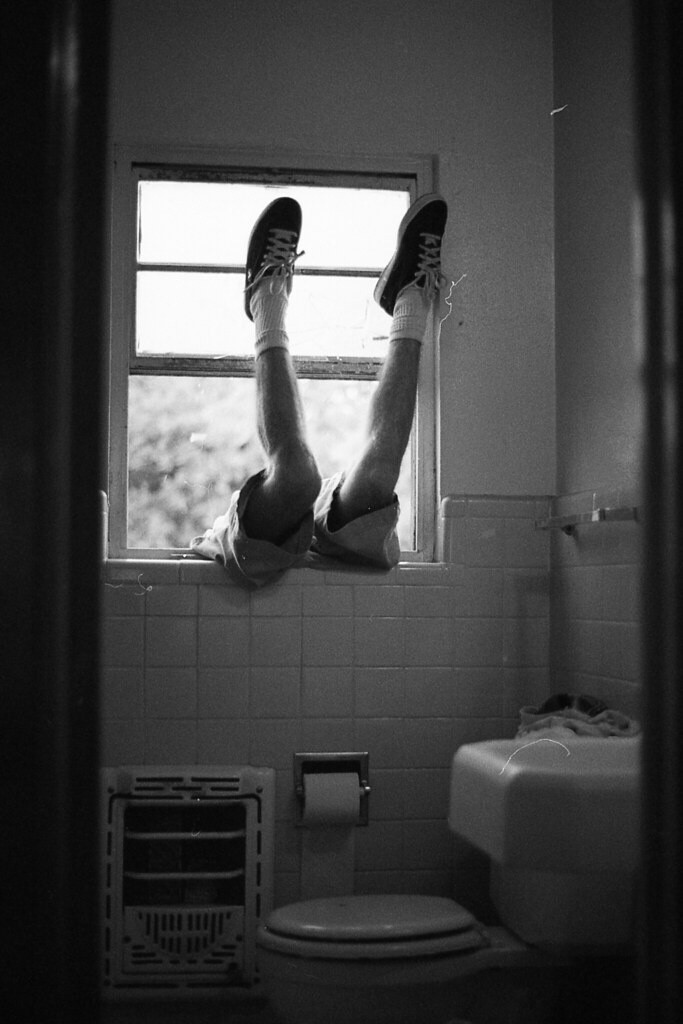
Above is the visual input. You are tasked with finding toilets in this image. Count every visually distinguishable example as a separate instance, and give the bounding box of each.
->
[256,732,646,1021]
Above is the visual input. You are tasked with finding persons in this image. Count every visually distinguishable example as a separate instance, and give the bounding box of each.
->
[189,193,455,590]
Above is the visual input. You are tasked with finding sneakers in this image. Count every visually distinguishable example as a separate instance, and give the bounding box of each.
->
[373,193,448,317]
[243,196,306,322]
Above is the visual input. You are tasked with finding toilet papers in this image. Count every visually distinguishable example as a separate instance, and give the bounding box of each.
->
[302,769,366,829]
